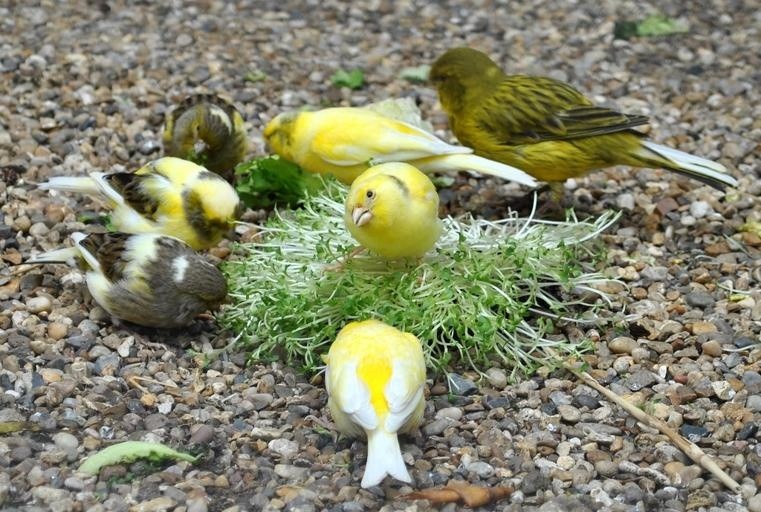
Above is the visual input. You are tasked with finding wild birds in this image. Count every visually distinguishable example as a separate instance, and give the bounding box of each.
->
[341,164,443,265]
[38,151,246,248]
[432,48,741,213]
[161,90,248,183]
[33,233,232,333]
[264,103,542,190]
[327,317,432,489]
